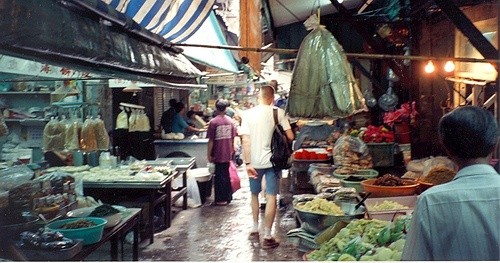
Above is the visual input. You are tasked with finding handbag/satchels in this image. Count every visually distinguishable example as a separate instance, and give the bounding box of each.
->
[229,160,240,193]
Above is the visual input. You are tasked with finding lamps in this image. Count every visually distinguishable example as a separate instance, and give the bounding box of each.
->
[122,80,143,93]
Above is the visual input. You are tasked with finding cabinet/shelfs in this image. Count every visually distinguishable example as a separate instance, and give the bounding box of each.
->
[0,90,81,121]
[154,139,209,168]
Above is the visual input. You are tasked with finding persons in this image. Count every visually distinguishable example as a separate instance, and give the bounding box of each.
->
[399,105,500,262]
[206,99,238,206]
[157,97,253,140]
[267,80,285,111]
[238,85,294,249]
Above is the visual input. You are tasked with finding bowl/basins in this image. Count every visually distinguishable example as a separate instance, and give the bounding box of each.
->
[65,205,127,229]
[294,165,421,235]
[0,215,40,239]
[44,217,107,245]
[191,167,213,182]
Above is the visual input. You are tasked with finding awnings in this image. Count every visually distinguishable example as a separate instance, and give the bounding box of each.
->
[0,0,216,92]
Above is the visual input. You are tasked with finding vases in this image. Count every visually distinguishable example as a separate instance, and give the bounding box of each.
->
[398,144,411,164]
[368,146,394,166]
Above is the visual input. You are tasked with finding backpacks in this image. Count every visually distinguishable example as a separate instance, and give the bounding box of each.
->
[271,109,292,169]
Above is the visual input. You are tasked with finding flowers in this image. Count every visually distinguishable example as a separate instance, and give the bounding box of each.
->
[384,101,416,143]
[361,125,393,143]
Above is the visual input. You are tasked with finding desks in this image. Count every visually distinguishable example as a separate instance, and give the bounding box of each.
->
[3,204,141,262]
[171,171,188,211]
[83,172,176,244]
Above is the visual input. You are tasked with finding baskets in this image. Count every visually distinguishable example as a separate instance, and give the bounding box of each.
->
[416,177,435,195]
[332,169,378,179]
[361,177,420,196]
[341,177,370,193]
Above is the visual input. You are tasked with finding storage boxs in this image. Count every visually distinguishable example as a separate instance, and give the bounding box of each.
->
[292,147,432,221]
[15,239,82,261]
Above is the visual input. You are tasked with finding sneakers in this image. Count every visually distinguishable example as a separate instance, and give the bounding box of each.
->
[263,237,279,248]
[248,232,260,241]
